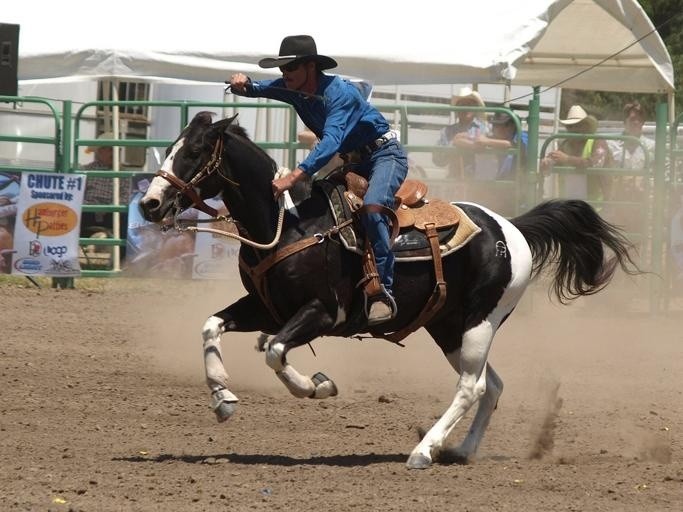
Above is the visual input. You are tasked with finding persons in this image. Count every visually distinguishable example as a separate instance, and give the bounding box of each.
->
[71,132,135,268]
[603,103,657,198]
[539,103,610,198]
[431,87,493,180]
[472,106,530,182]
[228,34,410,328]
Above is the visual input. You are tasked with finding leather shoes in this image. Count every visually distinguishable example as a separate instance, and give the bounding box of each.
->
[368,292,393,324]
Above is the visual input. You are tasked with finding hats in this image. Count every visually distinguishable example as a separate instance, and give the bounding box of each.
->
[451,87,486,118]
[488,112,513,124]
[258,35,338,71]
[556,105,598,134]
[85,132,114,153]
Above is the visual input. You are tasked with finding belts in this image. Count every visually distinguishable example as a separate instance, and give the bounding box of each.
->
[339,130,397,163]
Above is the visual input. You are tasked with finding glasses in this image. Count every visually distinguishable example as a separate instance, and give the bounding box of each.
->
[278,60,305,72]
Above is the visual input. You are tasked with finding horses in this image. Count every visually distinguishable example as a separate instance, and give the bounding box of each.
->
[137,111,667,469]
